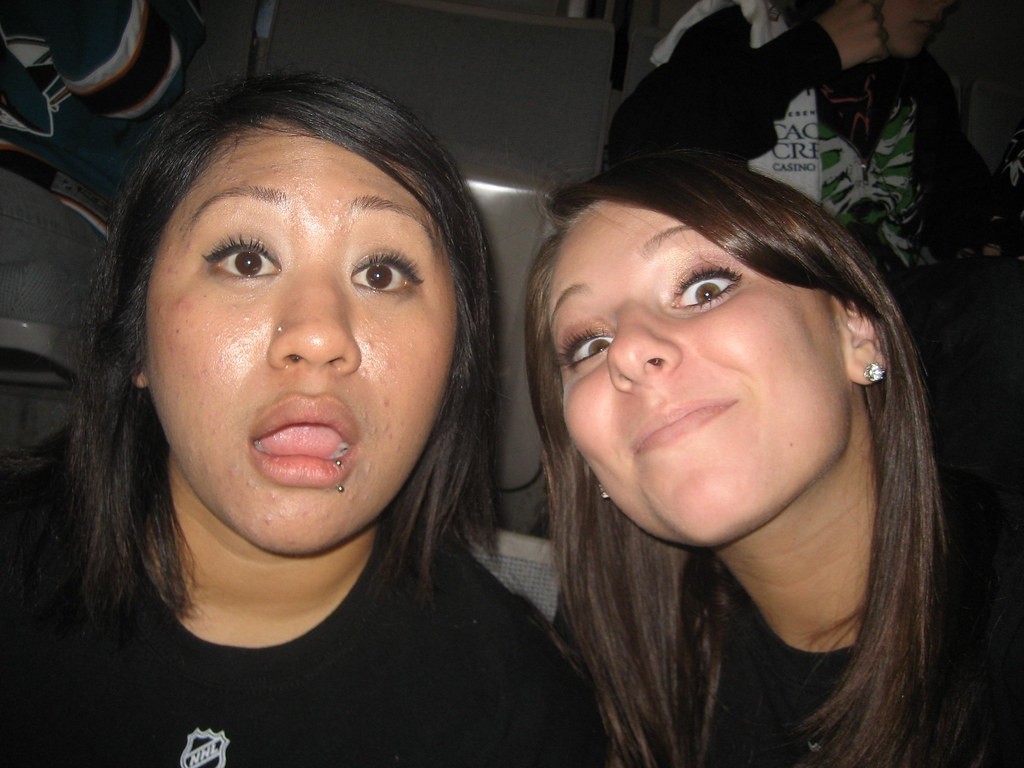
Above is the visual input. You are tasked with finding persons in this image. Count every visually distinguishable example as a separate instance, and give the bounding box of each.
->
[610,0,1023,487]
[1,69,609,768]
[526,157,1023,768]
